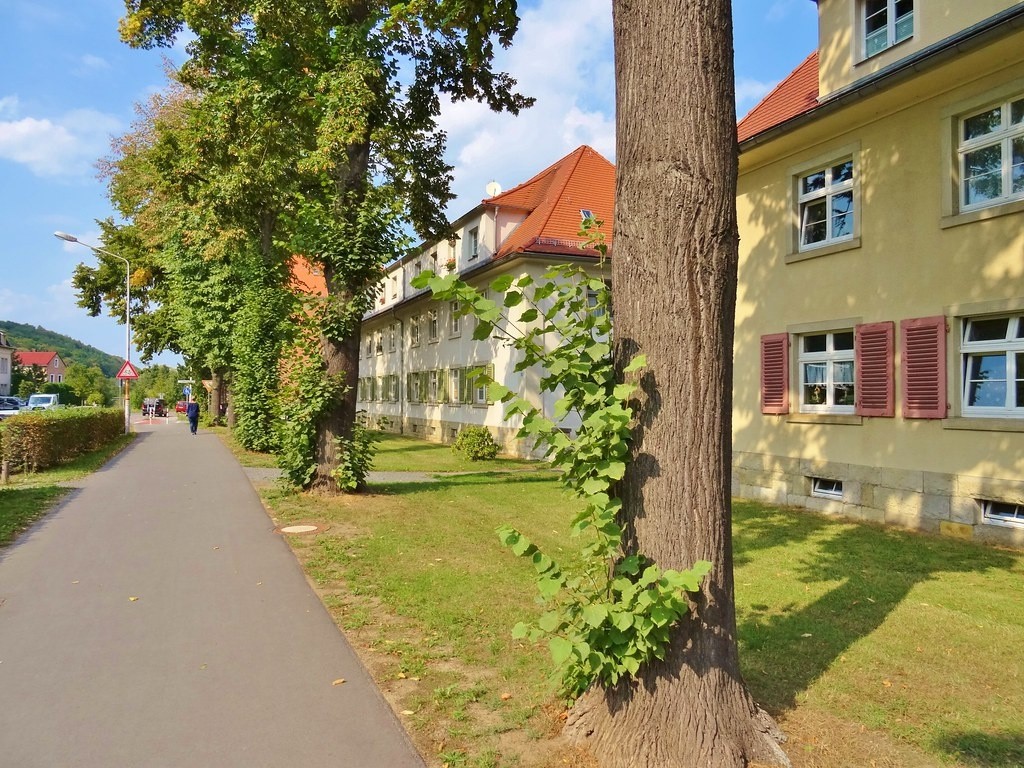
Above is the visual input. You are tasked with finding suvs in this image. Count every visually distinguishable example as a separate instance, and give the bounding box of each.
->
[176,401,186,412]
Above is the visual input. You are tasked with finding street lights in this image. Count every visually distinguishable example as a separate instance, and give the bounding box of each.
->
[53,231,130,435]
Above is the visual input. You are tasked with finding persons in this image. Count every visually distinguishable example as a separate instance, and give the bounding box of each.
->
[185,395,200,435]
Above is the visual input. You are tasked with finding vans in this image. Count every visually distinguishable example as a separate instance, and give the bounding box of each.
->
[27,393,58,412]
[143,398,167,417]
[0,397,26,410]
